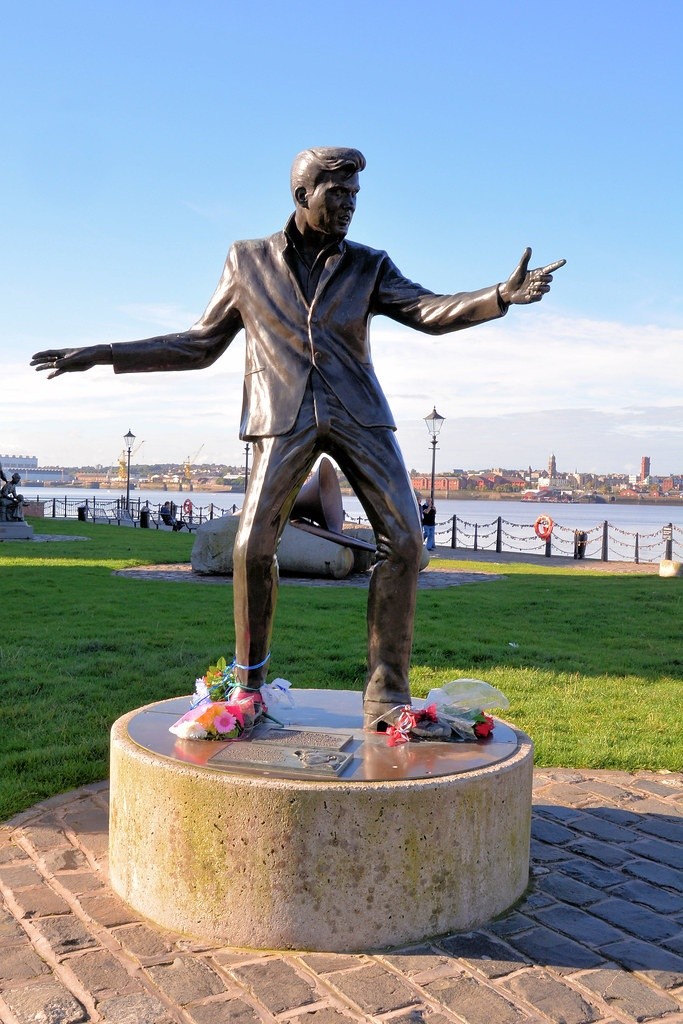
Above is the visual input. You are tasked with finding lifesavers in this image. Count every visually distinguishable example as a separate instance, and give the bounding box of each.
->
[183,498,193,513]
[533,516,554,539]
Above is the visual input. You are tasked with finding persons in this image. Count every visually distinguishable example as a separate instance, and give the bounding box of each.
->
[0,466,25,523]
[417,496,437,551]
[28,148,566,737]
[160,502,185,533]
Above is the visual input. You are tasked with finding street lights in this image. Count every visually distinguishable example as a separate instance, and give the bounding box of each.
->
[423,406,448,507]
[123,429,137,510]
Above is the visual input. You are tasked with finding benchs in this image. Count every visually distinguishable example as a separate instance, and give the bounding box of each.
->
[87,507,117,525]
[182,515,208,533]
[111,508,140,528]
[150,512,185,533]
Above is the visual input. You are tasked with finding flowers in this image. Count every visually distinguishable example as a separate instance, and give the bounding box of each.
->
[421,703,494,741]
[190,656,235,709]
[169,694,284,742]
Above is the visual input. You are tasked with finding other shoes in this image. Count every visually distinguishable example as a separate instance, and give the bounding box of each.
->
[427,549,434,551]
